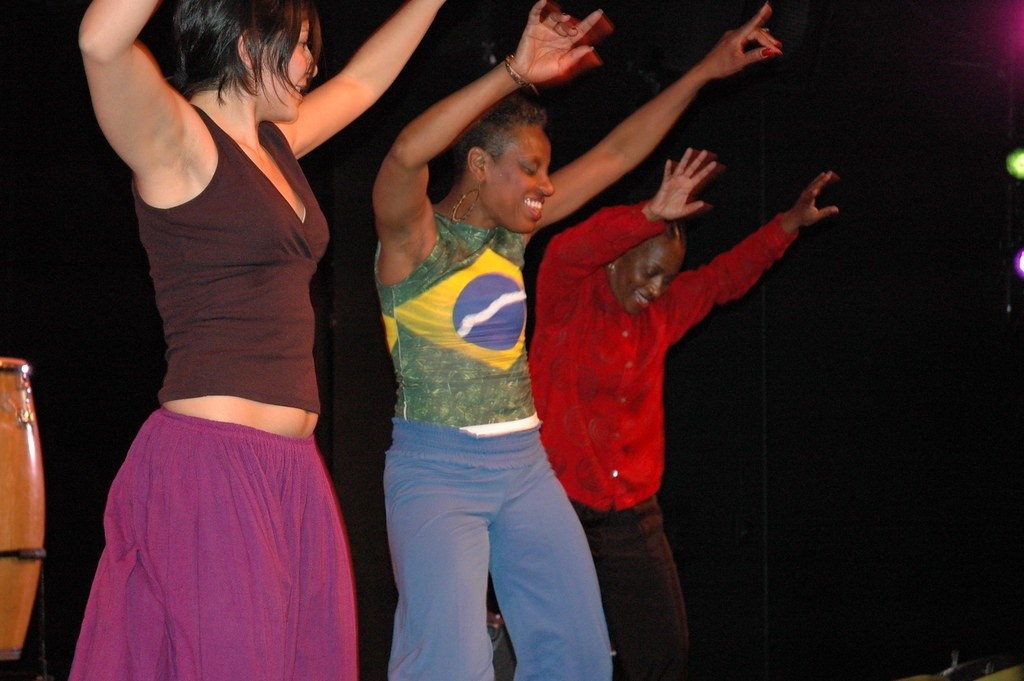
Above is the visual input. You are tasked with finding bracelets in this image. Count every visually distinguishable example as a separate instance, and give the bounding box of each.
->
[504,54,541,97]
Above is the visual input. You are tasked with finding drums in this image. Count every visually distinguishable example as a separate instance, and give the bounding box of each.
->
[1,356,50,663]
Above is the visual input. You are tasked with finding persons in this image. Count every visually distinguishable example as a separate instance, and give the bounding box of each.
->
[68,0,448,681]
[528,145,843,681]
[370,0,787,681]
